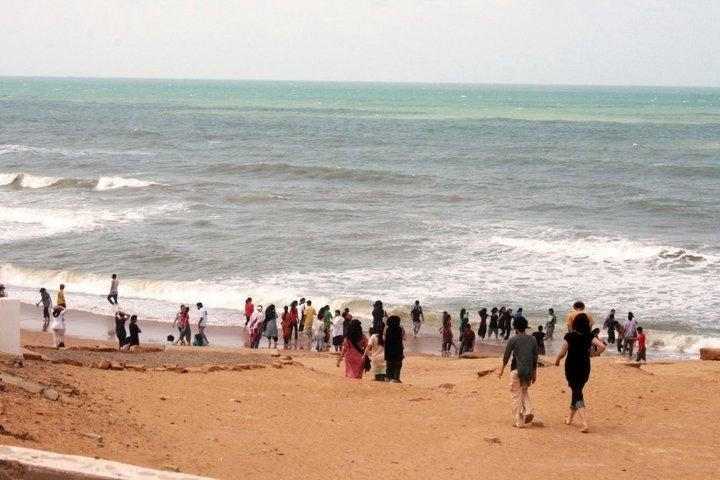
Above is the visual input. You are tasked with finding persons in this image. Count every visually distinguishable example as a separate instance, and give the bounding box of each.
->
[383,315,406,382]
[591,309,645,362]
[128,315,142,346]
[57,284,67,309]
[115,311,130,349]
[51,306,67,348]
[336,319,368,379]
[458,308,475,355]
[361,321,387,381]
[478,306,522,341]
[534,325,549,355]
[410,299,424,338]
[107,276,119,305]
[556,313,606,433]
[544,308,557,339]
[372,300,388,333]
[172,302,209,344]
[313,305,352,353]
[498,316,539,429]
[440,312,459,356]
[38,288,53,325]
[244,297,317,349]
[567,302,594,332]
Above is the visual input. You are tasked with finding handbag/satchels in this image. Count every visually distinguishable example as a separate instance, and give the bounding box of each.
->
[365,356,372,373]
